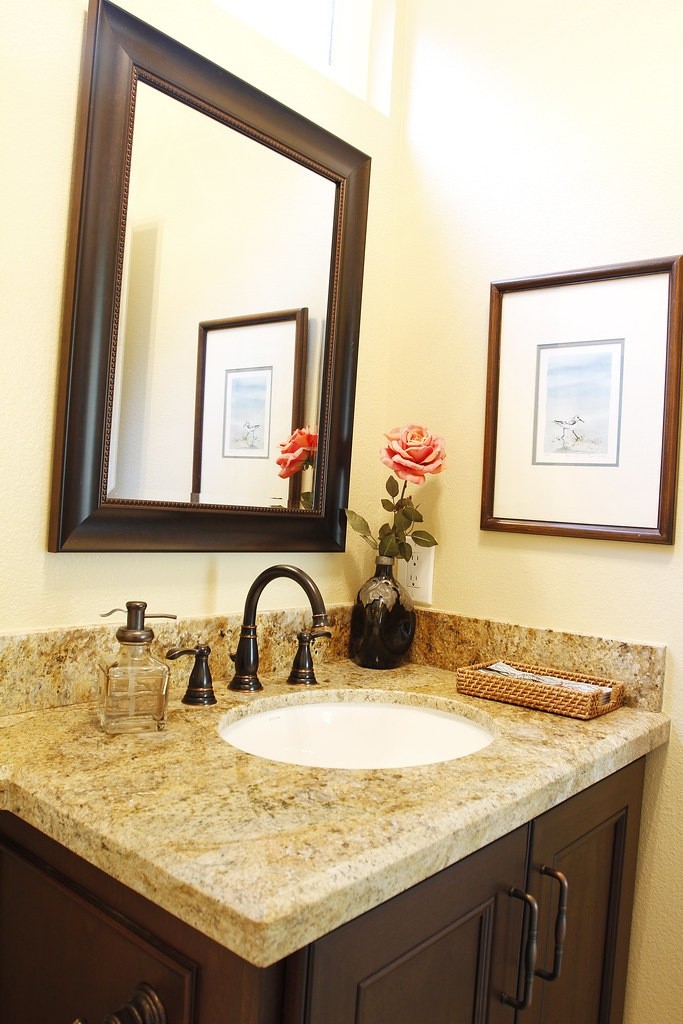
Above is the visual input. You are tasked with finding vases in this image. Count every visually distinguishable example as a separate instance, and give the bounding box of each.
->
[349,556,417,669]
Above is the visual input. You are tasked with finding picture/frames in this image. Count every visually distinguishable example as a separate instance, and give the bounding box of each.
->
[480,255,683,546]
[191,307,308,509]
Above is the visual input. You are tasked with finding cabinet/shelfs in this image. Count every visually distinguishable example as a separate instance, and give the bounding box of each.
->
[283,754,648,1024]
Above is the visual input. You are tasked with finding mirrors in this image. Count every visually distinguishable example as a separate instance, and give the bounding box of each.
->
[48,1,372,552]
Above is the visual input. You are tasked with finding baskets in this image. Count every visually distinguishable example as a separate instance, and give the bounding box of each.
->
[456,660,625,719]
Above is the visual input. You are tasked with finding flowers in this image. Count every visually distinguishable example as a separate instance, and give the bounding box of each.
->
[344,425,446,563]
[271,425,319,510]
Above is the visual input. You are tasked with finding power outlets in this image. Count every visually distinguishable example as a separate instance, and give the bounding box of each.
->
[397,535,436,604]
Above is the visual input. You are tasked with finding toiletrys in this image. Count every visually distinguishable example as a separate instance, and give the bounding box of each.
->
[96,601,177,734]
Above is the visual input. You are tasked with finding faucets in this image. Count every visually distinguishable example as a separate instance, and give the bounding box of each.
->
[226,564,330,692]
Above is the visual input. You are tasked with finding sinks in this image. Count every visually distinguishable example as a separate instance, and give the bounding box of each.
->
[209,688,503,769]
